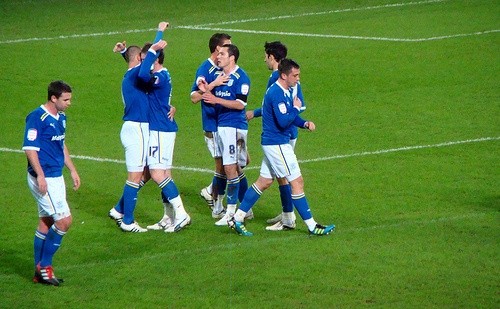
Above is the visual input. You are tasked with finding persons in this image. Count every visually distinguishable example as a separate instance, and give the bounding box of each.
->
[22,80,82,285]
[106,21,177,233]
[112,39,192,233]
[189,33,250,214]
[196,44,254,225]
[226,59,336,236]
[246,40,306,231]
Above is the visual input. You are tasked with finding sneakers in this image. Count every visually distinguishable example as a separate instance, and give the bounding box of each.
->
[308,224,335,236]
[120,219,148,232]
[147,215,174,230]
[211,207,227,219]
[227,217,254,236]
[200,188,215,211]
[33,276,63,287]
[164,213,191,234]
[265,220,296,231]
[266,214,283,223]
[108,208,125,221]
[214,213,234,225]
[244,211,254,220]
[36,260,60,286]
